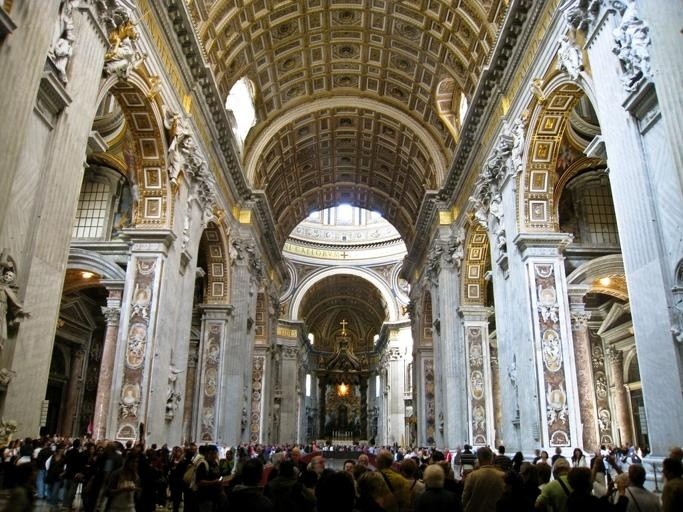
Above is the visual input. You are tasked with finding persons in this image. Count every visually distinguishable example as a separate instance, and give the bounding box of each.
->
[1,438,683,512]
